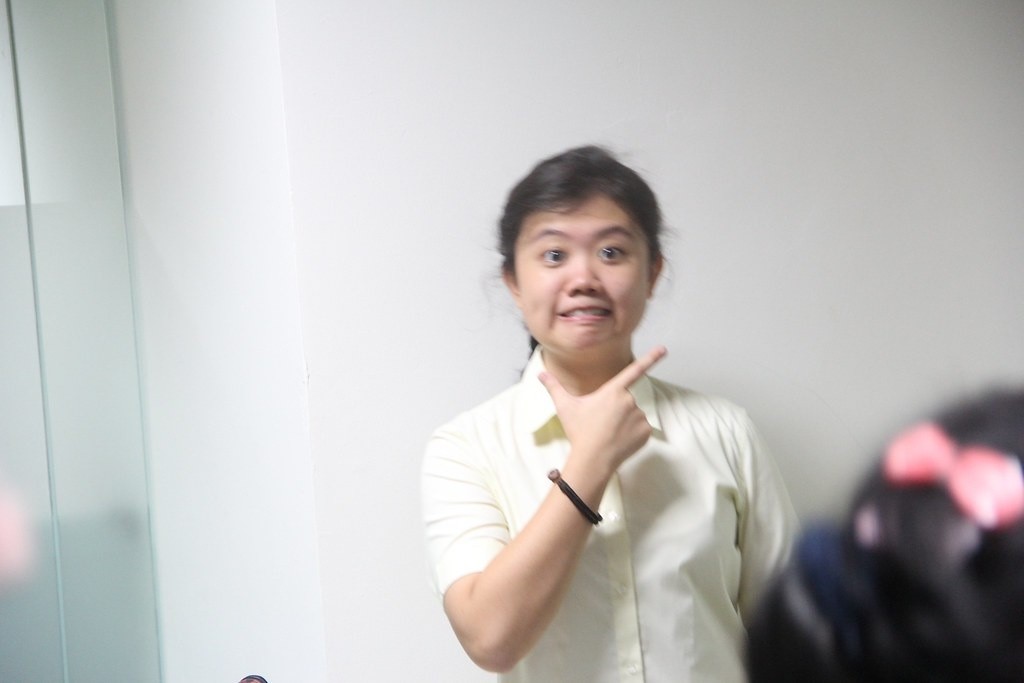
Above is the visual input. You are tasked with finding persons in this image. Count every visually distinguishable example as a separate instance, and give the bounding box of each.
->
[422,145,803,682]
[744,389,1024,680]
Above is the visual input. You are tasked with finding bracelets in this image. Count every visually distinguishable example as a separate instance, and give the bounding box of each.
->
[548,471,603,526]
